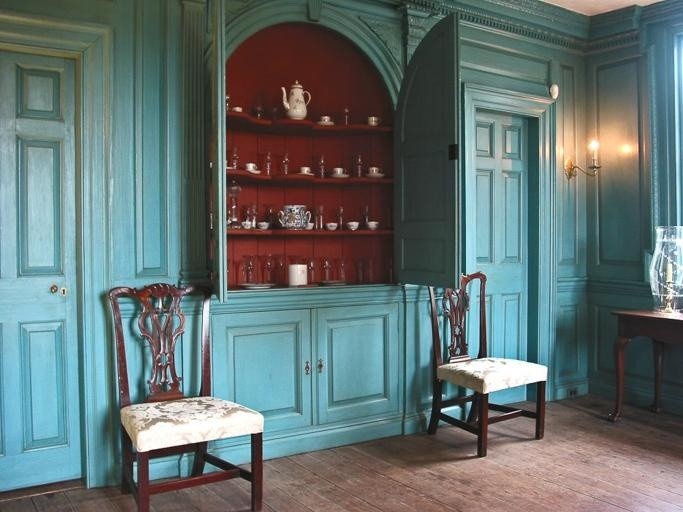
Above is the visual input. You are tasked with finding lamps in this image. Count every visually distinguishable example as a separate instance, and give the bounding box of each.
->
[561,133,606,185]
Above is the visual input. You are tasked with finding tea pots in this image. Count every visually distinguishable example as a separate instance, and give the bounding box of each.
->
[280,79,312,120]
[278,204,311,229]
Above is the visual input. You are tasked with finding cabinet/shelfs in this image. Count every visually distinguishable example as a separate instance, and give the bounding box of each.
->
[404,298,470,437]
[213,297,407,473]
[223,96,401,294]
[111,304,213,483]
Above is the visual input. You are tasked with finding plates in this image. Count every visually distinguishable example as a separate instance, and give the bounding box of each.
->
[366,173,384,178]
[317,121,336,126]
[239,283,276,290]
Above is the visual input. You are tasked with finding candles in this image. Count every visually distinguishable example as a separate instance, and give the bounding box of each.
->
[664,256,675,283]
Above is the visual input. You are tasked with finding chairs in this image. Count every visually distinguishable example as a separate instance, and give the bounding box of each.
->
[426,267,549,461]
[104,281,266,511]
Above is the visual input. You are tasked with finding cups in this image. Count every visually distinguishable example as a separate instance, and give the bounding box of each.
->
[232,107,242,113]
[368,167,381,174]
[241,222,378,230]
[367,116,381,127]
[241,254,349,285]
[320,116,331,123]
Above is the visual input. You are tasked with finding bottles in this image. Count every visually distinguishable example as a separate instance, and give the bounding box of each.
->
[243,206,257,229]
[335,205,345,230]
[360,205,369,230]
[229,146,366,178]
[225,180,241,231]
[344,105,352,125]
[316,205,325,228]
[253,96,265,119]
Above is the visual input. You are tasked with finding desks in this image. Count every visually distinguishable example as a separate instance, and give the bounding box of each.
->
[603,304,683,421]
